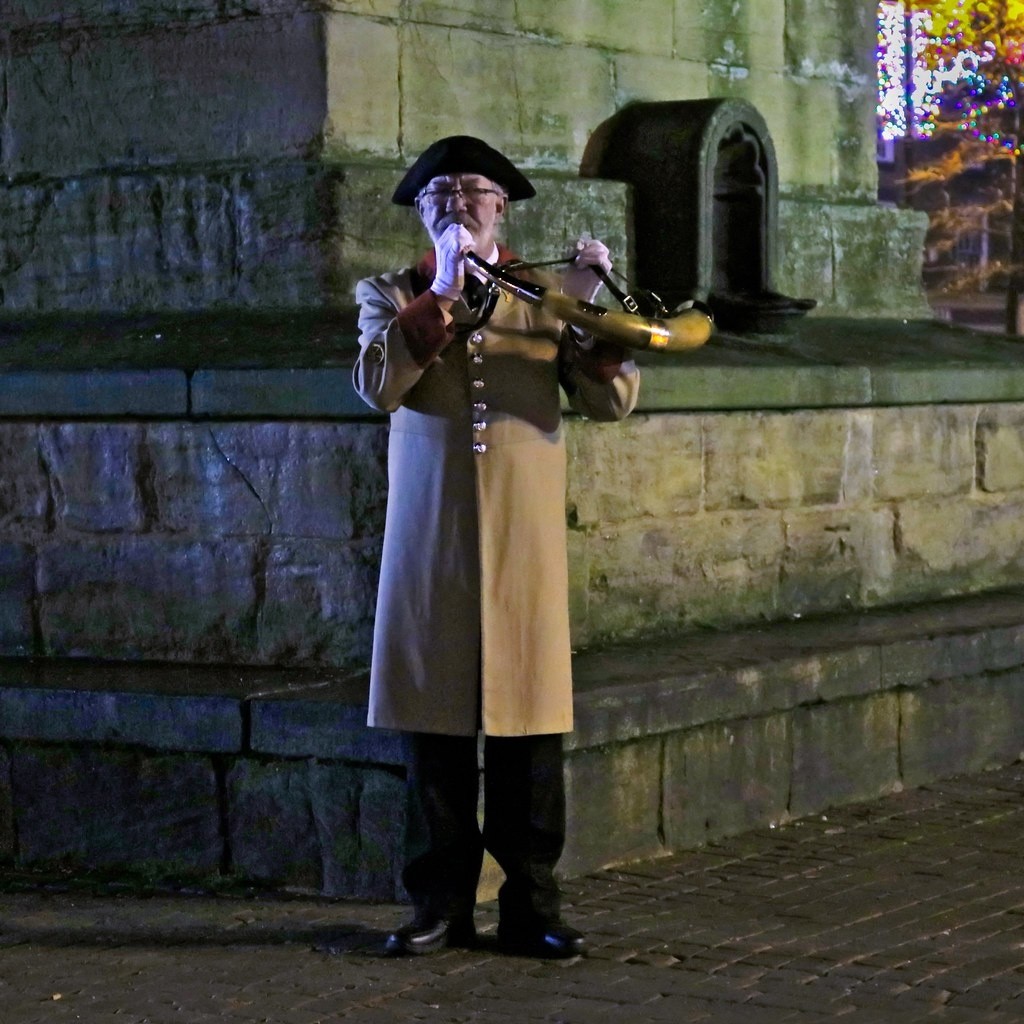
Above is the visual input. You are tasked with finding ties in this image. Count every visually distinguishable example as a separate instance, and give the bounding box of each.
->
[464,272,482,308]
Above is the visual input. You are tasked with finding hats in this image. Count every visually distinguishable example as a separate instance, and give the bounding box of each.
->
[391,136,536,207]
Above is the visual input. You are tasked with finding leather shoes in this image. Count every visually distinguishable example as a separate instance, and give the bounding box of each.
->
[386,911,477,955]
[496,923,586,957]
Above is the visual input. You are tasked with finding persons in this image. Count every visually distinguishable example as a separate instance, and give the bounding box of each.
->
[352,134,641,958]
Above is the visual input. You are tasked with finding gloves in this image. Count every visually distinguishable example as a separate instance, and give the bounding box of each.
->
[430,223,478,302]
[562,239,614,305]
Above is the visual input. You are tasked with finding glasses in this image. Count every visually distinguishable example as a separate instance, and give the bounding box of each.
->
[421,186,502,205]
